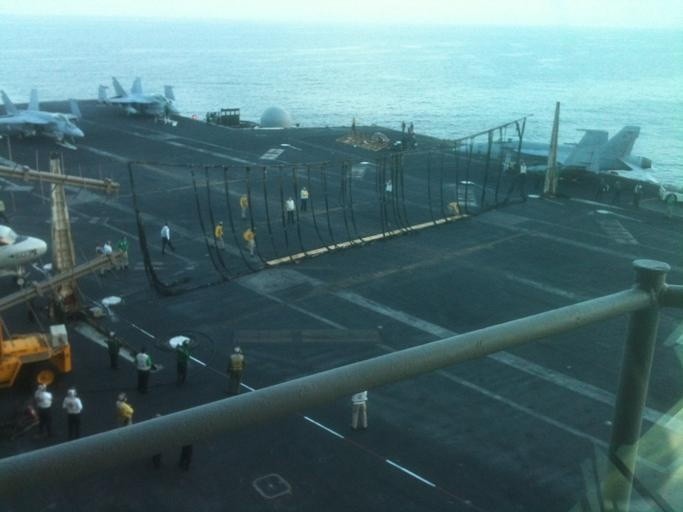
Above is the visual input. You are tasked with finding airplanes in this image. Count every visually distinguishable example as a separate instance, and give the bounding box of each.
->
[450,123,660,190]
[99,75,178,122]
[0,88,85,154]
[0,201,48,290]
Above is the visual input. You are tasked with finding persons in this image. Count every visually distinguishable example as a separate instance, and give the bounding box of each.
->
[34,381,53,433]
[178,446,193,470]
[612,179,624,204]
[148,411,165,466]
[631,182,645,209]
[118,234,129,270]
[103,240,112,267]
[59,390,84,439]
[133,344,150,393]
[160,220,175,254]
[597,174,607,196]
[115,389,133,426]
[285,196,295,223]
[214,220,224,251]
[243,225,258,256]
[347,389,370,430]
[238,190,248,220]
[298,185,310,210]
[105,331,120,365]
[93,242,105,278]
[175,342,186,386]
[226,345,244,396]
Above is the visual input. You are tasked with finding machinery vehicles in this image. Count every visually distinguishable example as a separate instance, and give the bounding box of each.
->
[0,243,129,388]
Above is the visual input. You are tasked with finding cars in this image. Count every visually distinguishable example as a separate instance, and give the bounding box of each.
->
[659,181,683,207]
